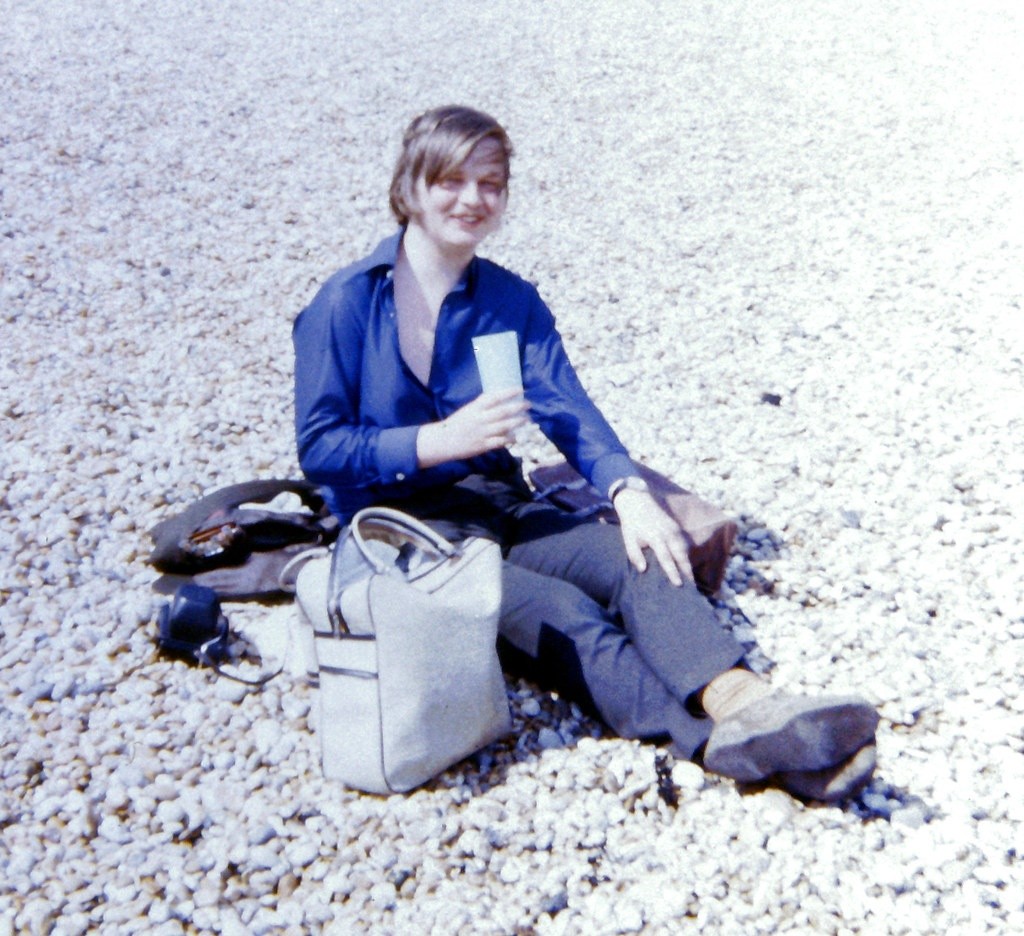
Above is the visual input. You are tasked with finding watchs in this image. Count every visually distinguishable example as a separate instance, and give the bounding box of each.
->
[611,476,649,504]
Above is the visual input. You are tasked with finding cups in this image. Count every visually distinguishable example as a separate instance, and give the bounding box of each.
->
[473,330,530,446]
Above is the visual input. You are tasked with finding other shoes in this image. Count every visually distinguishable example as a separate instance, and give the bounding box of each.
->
[701,688,877,804]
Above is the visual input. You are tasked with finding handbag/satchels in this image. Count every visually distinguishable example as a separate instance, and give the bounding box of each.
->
[278,506,515,796]
[149,481,330,571]
[529,453,736,600]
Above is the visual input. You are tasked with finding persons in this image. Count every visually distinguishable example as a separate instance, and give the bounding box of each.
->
[291,104,882,803]
